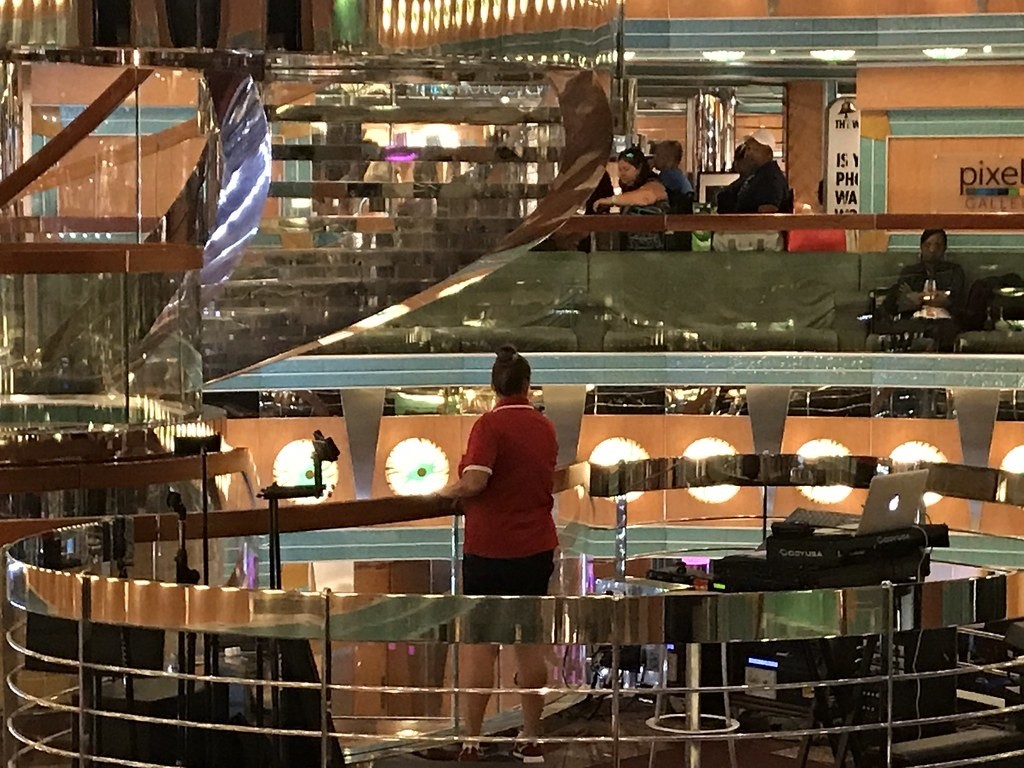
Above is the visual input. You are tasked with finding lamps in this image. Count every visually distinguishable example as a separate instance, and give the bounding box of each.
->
[998,443,1024,510]
[793,437,854,505]
[588,436,652,504]
[683,437,743,504]
[273,438,339,506]
[385,436,448,498]
[888,440,951,510]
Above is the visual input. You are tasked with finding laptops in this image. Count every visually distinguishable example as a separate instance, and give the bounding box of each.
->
[813,469,930,539]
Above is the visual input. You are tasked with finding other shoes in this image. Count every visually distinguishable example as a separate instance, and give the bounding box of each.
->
[513,737,545,763]
[458,742,480,763]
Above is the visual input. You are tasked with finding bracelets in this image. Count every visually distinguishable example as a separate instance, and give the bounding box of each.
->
[611,195,617,204]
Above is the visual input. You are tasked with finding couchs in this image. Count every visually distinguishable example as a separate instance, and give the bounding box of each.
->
[587,251,860,354]
[859,250,1024,356]
[318,250,588,355]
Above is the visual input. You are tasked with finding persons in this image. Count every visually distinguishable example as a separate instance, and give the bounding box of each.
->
[884,227,968,354]
[736,129,788,215]
[715,143,753,216]
[429,345,559,764]
[649,141,694,207]
[590,145,672,251]
[316,131,523,282]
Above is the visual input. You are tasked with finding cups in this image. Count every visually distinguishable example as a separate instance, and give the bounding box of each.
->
[923,279,936,300]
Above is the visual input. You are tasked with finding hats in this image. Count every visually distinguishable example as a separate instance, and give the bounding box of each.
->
[744,129,776,153]
